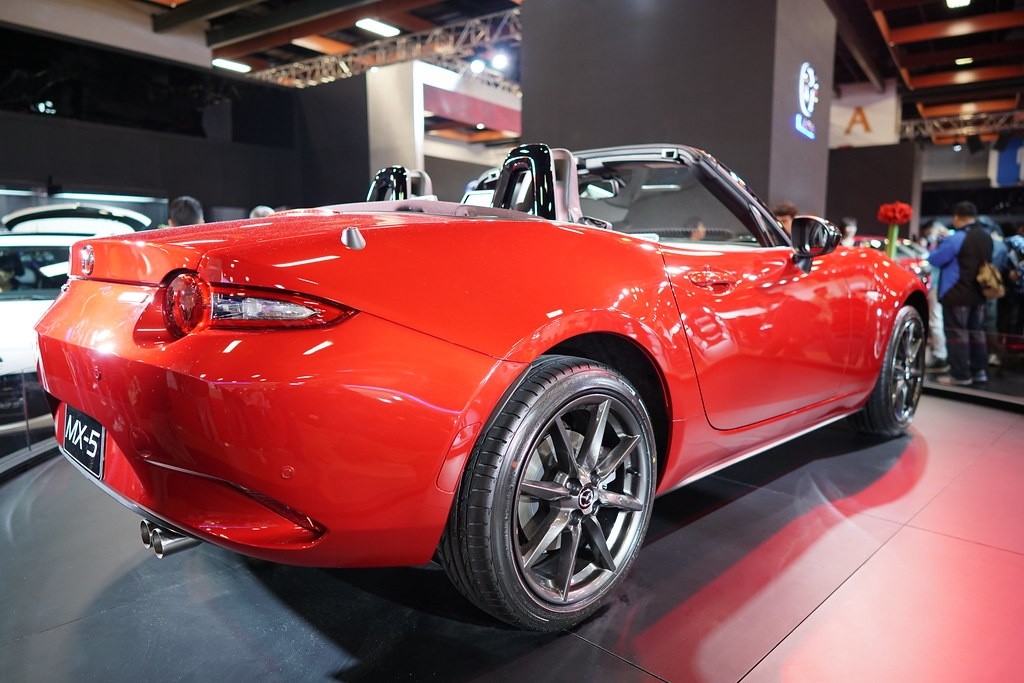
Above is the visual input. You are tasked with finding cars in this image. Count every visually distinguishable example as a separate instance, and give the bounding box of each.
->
[0,204,153,446]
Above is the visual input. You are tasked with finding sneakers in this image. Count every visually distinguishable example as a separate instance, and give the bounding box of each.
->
[936,373,972,385]
[972,369,988,381]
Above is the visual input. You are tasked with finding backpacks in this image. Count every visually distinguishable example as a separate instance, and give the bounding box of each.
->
[975,260,1004,298]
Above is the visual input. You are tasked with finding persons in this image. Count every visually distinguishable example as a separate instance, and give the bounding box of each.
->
[0,257,34,293]
[926,202,995,385]
[913,215,1024,348]
[681,217,707,242]
[773,201,797,237]
[975,215,1009,366]
[836,217,860,247]
[169,195,205,227]
[922,219,957,375]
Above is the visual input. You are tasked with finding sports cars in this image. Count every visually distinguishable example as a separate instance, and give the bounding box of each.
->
[36,141,931,636]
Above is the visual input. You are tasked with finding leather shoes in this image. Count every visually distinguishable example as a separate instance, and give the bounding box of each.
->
[924,358,951,373]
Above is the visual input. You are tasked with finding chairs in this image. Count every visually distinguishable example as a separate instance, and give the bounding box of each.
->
[365,164,433,204]
[488,140,583,224]
